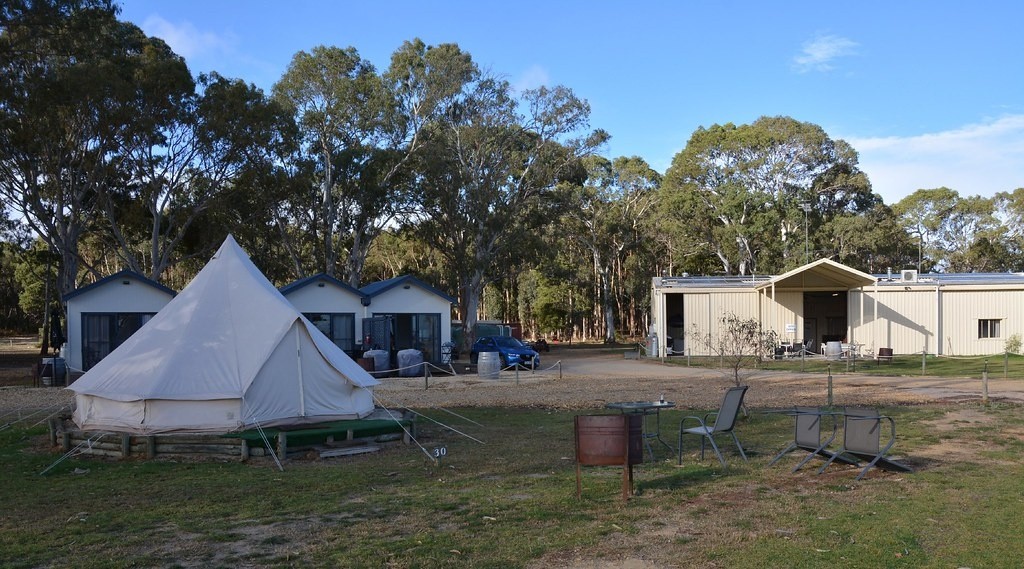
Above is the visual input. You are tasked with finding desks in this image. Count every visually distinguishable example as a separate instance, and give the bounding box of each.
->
[781,344,791,358]
[605,399,676,459]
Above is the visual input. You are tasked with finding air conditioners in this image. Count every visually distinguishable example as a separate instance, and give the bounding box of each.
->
[902,269,918,283]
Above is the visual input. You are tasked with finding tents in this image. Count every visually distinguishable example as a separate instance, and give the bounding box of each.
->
[63,232,383,434]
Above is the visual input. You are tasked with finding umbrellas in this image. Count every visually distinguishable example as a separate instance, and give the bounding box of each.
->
[50,308,66,353]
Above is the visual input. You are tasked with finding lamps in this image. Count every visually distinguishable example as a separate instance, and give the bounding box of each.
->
[319,282,324,287]
[122,279,130,285]
[404,285,411,289]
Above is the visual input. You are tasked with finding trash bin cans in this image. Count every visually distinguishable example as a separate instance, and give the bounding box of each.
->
[775,348,784,359]
[646,337,659,357]
[478,351,501,380]
[42,358,65,386]
[826,341,842,361]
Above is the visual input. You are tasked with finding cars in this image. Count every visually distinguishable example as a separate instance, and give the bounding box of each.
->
[470,335,540,371]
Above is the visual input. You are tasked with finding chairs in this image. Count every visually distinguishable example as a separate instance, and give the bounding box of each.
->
[793,340,803,357]
[780,338,792,357]
[758,404,864,473]
[816,408,914,485]
[798,339,814,357]
[674,386,754,478]
